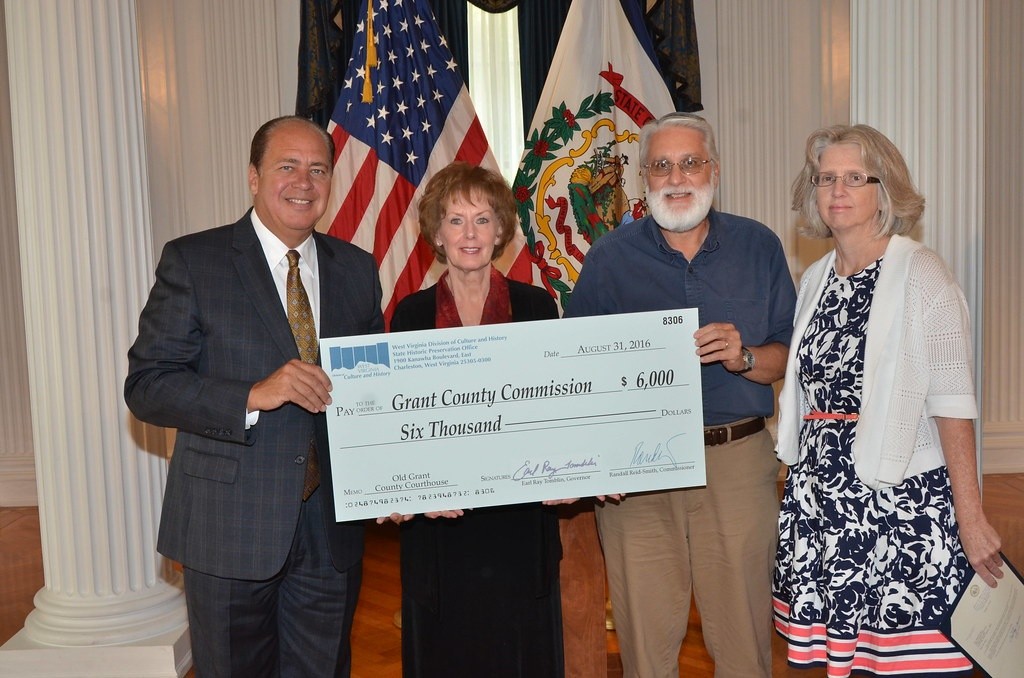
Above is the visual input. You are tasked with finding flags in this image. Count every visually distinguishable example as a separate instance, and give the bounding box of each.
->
[511,0,677,318]
[313,0,534,333]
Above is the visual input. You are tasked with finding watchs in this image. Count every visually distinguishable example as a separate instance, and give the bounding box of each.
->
[727,344,757,375]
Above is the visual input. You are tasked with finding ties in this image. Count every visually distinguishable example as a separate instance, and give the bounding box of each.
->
[287,248,322,500]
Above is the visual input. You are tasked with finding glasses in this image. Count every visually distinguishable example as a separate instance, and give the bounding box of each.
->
[644,158,713,177]
[811,172,881,187]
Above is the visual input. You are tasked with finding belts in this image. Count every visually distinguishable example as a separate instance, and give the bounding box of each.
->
[704,414,768,447]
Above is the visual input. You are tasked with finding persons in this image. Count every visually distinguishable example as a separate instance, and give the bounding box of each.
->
[769,121,1004,677]
[387,160,569,678]
[562,114,796,676]
[121,117,380,678]
